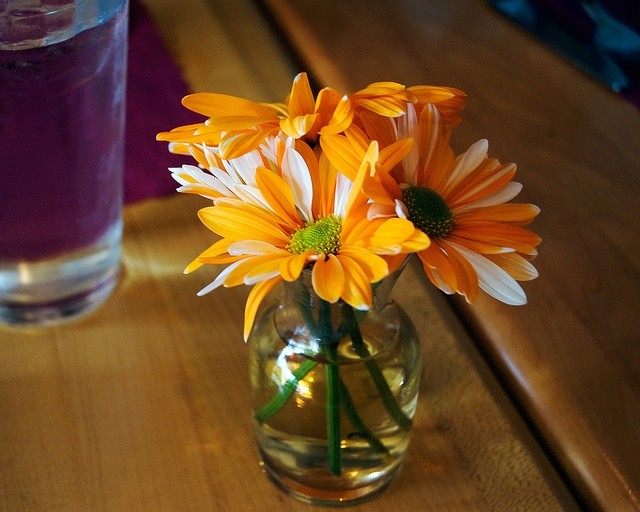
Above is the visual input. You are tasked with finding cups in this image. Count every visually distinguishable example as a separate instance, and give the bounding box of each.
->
[0,0,124,331]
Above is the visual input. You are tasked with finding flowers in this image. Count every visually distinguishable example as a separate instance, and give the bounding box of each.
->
[157,71,553,475]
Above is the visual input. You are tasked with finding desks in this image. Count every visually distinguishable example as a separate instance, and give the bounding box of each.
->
[0,0,640,512]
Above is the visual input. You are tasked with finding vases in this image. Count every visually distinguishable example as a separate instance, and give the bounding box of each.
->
[248,262,421,508]
